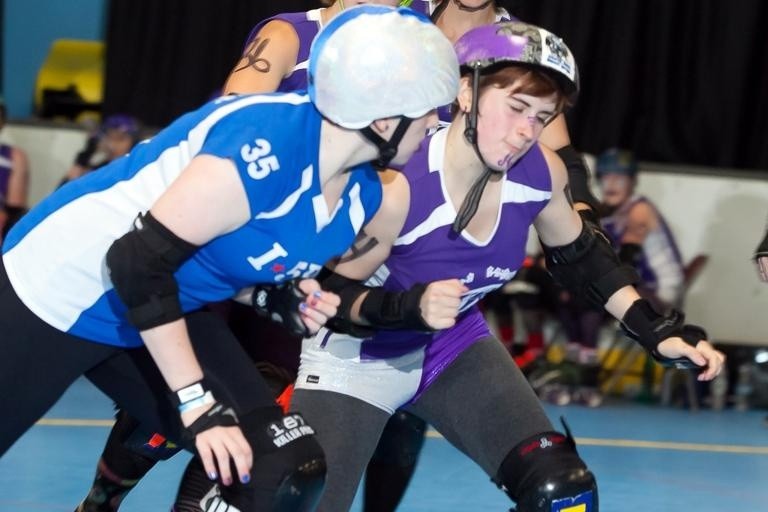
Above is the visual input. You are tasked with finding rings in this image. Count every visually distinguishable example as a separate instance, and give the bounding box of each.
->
[759,270,765,273]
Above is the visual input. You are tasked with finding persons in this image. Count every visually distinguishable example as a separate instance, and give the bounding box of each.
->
[286,21,724,512]
[77,0,410,510]
[537,146,684,399]
[487,270,550,380]
[57,116,142,193]
[361,0,599,512]
[2,141,28,233]
[753,227,768,283]
[1,0,457,512]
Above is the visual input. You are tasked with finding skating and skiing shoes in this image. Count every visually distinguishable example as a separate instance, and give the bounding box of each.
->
[533,359,603,408]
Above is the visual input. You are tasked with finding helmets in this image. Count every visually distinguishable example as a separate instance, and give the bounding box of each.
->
[596,149,636,178]
[99,116,139,139]
[308,6,458,130]
[454,20,579,100]
